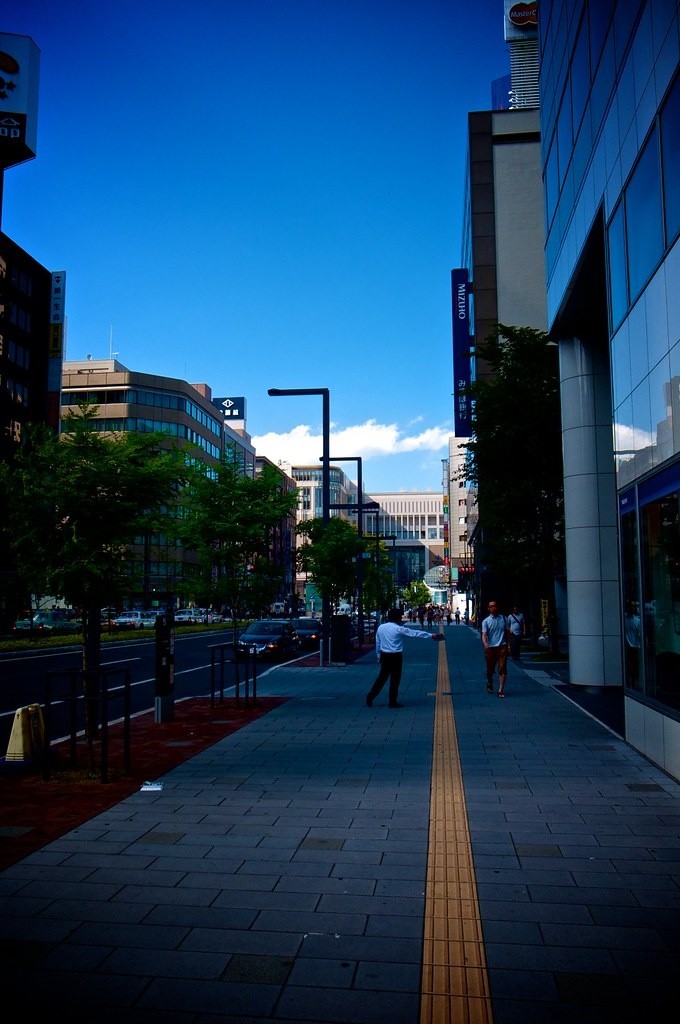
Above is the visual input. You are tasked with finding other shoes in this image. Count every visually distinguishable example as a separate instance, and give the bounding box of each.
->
[389,704,404,708]
[366,696,372,707]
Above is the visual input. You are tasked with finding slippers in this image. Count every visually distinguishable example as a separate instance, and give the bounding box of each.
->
[487,681,493,693]
[498,692,505,698]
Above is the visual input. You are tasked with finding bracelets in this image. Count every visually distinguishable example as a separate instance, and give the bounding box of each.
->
[508,646,510,648]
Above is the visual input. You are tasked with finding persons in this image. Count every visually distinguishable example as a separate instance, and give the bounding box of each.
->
[625,598,680,658]
[366,608,447,709]
[311,607,319,618]
[482,599,511,698]
[506,606,525,661]
[408,602,469,629]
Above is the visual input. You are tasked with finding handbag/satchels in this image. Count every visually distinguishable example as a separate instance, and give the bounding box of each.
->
[521,624,524,632]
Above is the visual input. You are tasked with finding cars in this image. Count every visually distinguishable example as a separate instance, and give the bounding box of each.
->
[112,608,237,628]
[298,607,306,615]
[291,618,323,651]
[336,607,350,615]
[12,611,80,630]
[238,620,302,662]
